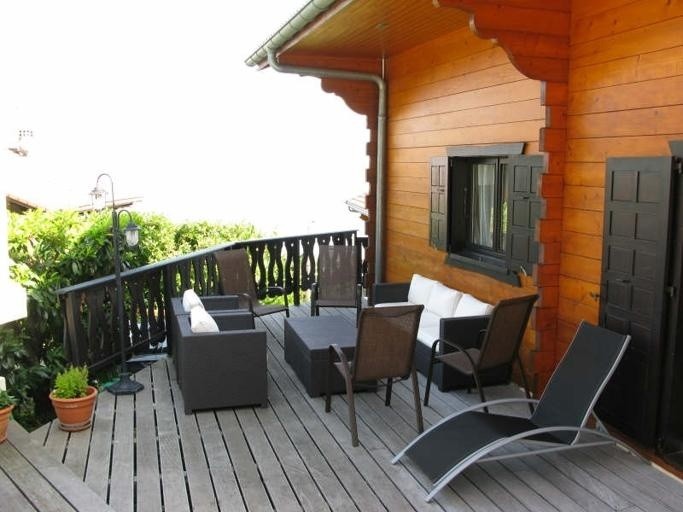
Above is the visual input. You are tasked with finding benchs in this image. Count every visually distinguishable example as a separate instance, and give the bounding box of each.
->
[367,273,514,392]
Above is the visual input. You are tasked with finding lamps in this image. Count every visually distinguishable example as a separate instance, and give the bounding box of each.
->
[117,207,138,247]
[89,174,117,211]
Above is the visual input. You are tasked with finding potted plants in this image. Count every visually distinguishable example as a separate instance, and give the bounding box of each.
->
[0,389,16,443]
[50,361,98,431]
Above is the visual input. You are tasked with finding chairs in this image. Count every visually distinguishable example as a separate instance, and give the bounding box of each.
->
[424,293,539,414]
[392,320,657,503]
[310,244,362,327]
[169,294,250,383]
[213,249,289,330]
[325,305,424,447]
[170,312,266,415]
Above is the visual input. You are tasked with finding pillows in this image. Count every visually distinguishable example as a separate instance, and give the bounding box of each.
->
[406,274,440,302]
[426,284,460,319]
[190,306,219,333]
[182,289,204,312]
[454,294,494,318]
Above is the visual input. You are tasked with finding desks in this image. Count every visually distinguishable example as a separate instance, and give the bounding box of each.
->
[284,315,378,397]
